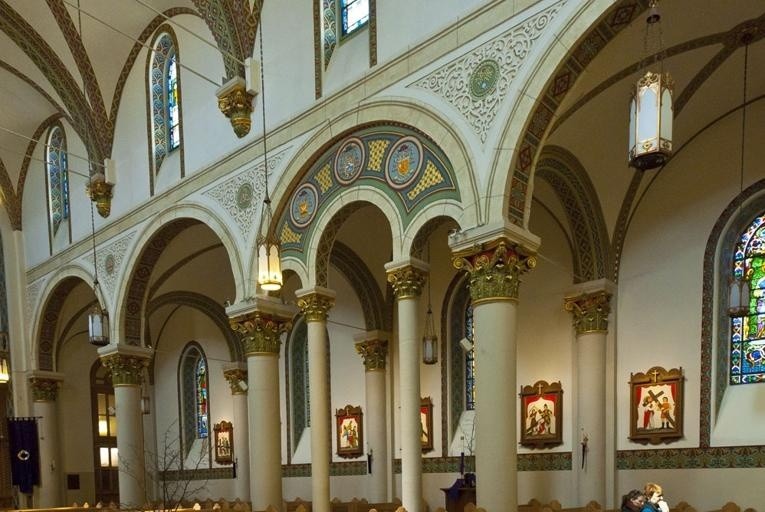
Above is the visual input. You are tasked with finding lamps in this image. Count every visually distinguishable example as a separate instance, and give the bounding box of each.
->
[256,0,283,292]
[421,239,439,366]
[88,1,108,345]
[628,1,675,170]
[726,30,752,318]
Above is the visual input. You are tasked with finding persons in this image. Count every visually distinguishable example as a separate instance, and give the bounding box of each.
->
[659,396,673,428]
[217,437,229,456]
[540,402,553,433]
[341,420,358,448]
[644,397,659,428]
[641,483,670,512]
[528,407,541,435]
[621,488,644,512]
